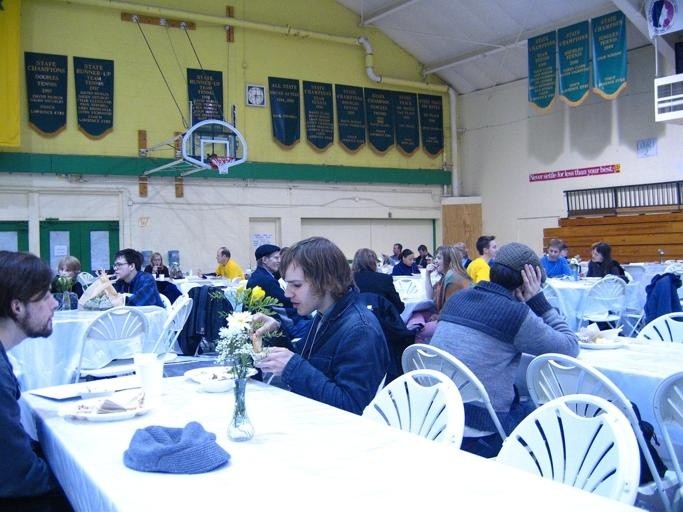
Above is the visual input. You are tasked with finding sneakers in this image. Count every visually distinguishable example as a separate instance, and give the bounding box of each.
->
[408,324,423,336]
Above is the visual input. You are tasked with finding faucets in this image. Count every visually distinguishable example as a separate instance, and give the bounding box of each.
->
[657,248,664,265]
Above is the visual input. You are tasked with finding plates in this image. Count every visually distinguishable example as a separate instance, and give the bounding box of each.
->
[179,276,223,284]
[182,365,257,393]
[578,334,626,349]
[546,277,601,284]
[72,399,149,422]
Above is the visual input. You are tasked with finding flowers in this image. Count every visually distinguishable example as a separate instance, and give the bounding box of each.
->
[58,270,73,291]
[657,247,665,255]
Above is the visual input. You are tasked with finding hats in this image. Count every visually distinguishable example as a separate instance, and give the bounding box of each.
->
[122,420,231,474]
[254,244,281,261]
[494,241,547,284]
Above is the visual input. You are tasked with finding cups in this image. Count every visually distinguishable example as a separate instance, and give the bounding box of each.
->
[151,272,165,279]
[133,352,170,398]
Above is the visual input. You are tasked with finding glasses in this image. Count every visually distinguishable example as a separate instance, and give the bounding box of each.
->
[112,262,128,270]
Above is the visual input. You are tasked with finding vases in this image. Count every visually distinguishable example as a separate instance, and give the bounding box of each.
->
[659,255,664,264]
[62,290,71,311]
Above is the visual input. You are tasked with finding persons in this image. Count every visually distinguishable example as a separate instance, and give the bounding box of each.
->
[205,247,245,280]
[243,236,391,416]
[381,237,579,344]
[104,248,165,307]
[587,241,629,336]
[0,250,60,512]
[144,252,170,278]
[351,248,406,314]
[429,242,578,459]
[51,255,84,310]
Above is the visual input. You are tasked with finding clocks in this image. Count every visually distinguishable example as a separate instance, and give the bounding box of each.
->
[246,84,266,107]
